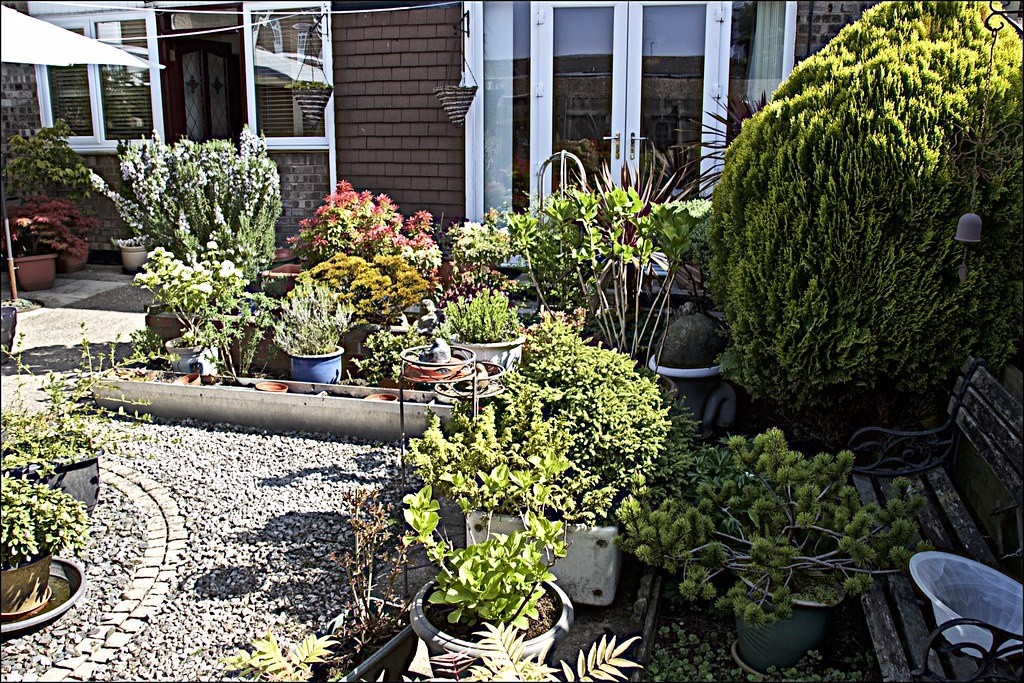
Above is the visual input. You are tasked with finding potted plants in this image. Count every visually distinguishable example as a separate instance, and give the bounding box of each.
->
[0,116,179,635]
[433,40,478,129]
[284,79,335,131]
[324,185,938,683]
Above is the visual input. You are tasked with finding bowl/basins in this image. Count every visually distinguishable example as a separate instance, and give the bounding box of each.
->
[173,373,200,385]
[909,551,1023,659]
[365,393,398,402]
[255,382,289,393]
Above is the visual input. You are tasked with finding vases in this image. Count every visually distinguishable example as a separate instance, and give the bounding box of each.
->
[261,263,302,298]
[272,249,300,265]
[165,335,218,375]
[289,344,345,383]
[107,366,414,404]
[119,240,147,273]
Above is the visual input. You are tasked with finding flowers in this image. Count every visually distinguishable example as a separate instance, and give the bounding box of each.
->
[87,120,608,355]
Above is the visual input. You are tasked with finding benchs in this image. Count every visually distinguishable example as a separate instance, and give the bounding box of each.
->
[833,352,1024,683]
